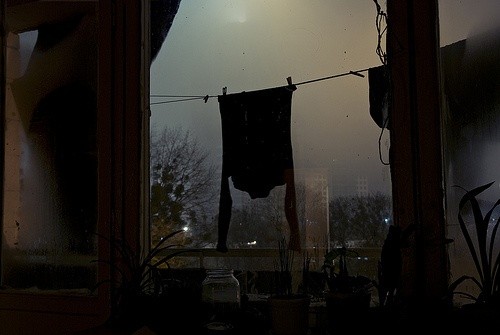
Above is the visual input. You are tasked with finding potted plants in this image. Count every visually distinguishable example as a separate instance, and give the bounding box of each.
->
[86,230,185,335]
[266,237,310,335]
[328,246,371,312]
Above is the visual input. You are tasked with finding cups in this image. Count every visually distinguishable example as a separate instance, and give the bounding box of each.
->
[202,269,240,330]
